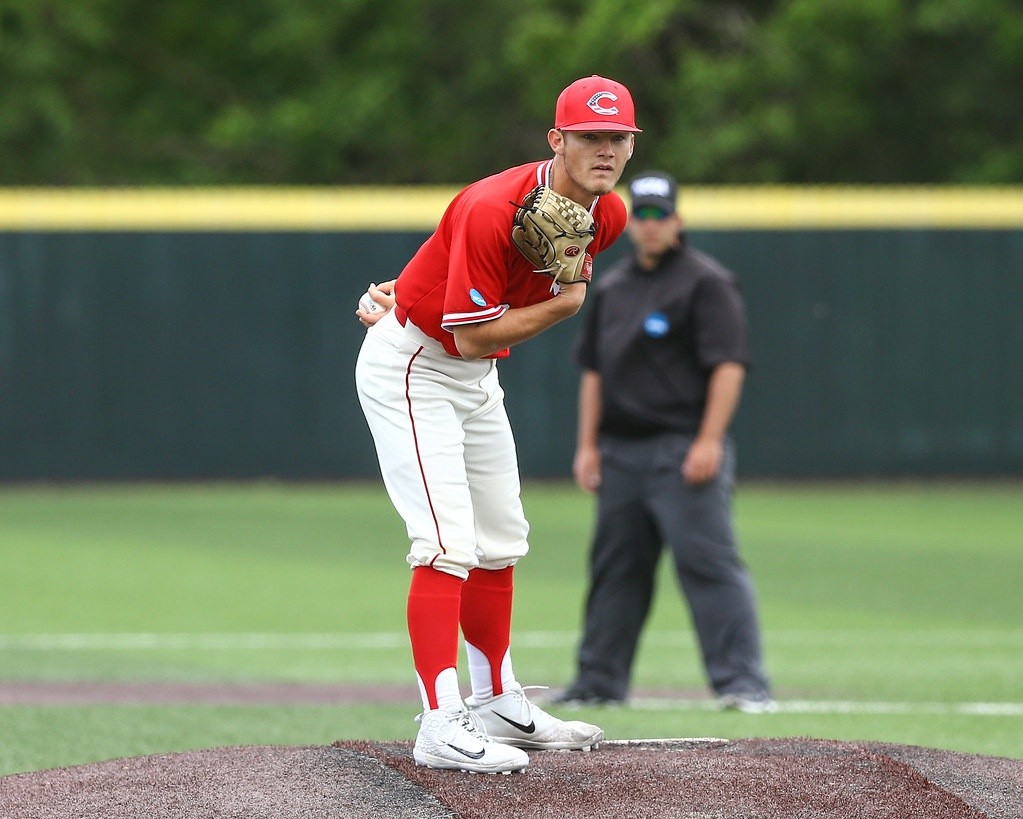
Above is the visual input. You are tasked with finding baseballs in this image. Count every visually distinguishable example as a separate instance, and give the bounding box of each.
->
[359,290,387,315]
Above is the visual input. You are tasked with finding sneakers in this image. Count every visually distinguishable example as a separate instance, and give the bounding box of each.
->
[412,705,529,776]
[464,683,604,752]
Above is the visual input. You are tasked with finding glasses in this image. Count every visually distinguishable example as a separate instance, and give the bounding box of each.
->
[632,206,668,220]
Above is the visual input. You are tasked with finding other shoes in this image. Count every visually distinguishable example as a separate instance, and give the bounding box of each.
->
[533,684,621,708]
[718,684,778,713]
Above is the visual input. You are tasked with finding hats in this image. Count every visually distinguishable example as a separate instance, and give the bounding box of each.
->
[554,75,643,133]
[628,169,677,215]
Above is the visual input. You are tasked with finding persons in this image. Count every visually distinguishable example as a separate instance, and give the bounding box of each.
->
[355,72,637,773]
[531,173,779,716]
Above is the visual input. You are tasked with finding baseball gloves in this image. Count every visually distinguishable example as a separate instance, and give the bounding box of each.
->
[506,183,600,295]
